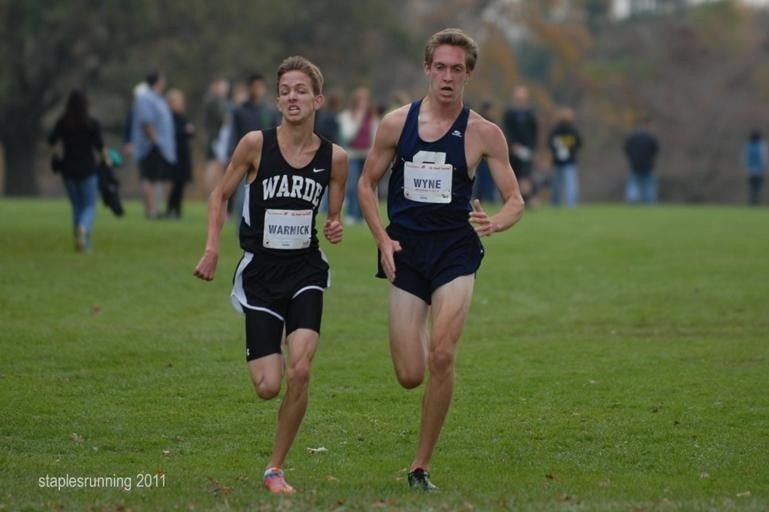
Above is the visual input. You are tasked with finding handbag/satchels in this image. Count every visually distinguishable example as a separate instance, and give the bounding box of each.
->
[96,165,125,217]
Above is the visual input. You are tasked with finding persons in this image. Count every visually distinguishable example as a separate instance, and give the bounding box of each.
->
[194,57,348,492]
[624,112,658,203]
[124,75,581,221]
[51,87,113,252]
[744,127,768,205]
[356,31,526,493]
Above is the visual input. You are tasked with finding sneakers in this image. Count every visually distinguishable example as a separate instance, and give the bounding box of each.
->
[261,467,298,495]
[143,208,181,222]
[406,467,439,491]
[69,226,88,252]
